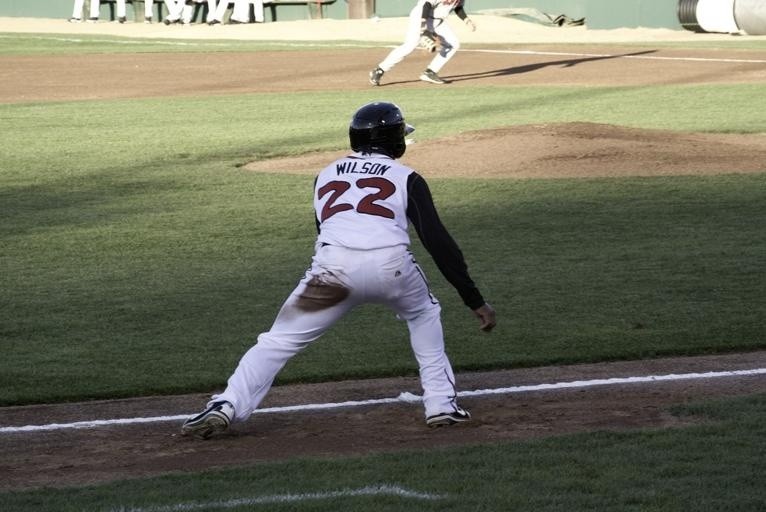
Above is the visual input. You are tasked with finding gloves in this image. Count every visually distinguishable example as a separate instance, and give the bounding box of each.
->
[467,21,477,32]
[476,303,496,329]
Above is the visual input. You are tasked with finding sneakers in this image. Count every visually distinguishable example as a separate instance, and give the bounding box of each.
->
[419,69,445,84]
[368,68,383,85]
[181,401,235,441]
[427,406,471,429]
[69,17,240,25]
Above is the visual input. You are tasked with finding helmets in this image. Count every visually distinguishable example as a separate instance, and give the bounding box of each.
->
[349,101,416,152]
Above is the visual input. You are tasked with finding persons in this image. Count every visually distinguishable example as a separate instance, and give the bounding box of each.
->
[66,1,264,27]
[368,1,478,87]
[181,102,500,437]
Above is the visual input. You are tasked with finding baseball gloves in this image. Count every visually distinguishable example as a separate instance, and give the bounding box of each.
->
[419,31,441,54]
